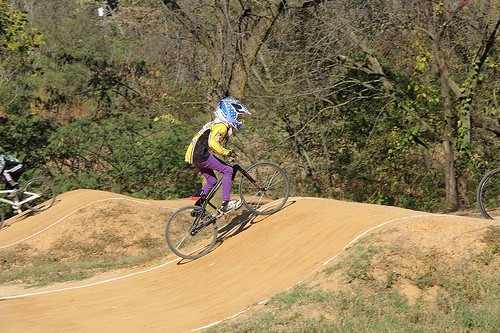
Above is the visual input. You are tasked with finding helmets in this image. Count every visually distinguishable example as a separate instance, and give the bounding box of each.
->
[214,98,250,129]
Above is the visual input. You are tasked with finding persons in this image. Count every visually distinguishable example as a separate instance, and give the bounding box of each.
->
[0,147,26,213]
[185,97,252,218]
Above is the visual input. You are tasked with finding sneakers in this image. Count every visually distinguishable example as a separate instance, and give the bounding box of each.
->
[192,209,207,216]
[221,200,241,212]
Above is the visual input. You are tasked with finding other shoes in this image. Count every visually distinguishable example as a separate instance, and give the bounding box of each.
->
[12,186,22,194]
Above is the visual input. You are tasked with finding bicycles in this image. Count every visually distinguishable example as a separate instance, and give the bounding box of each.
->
[164,148,290,260]
[476,167,500,220]
[0,178,56,228]
